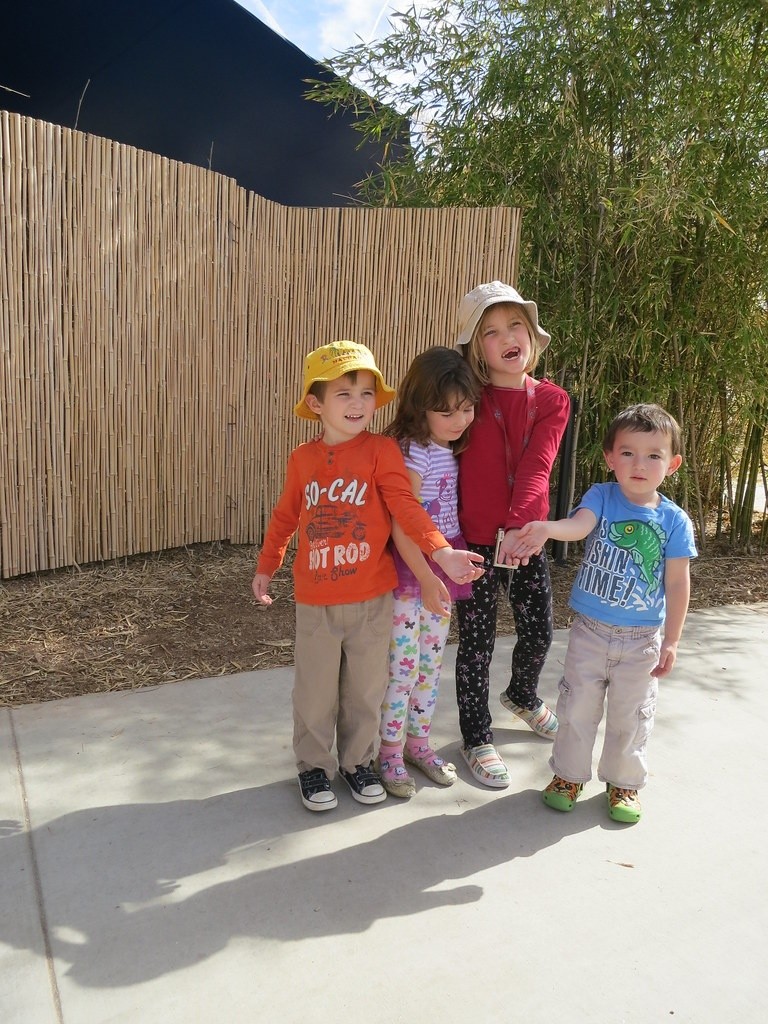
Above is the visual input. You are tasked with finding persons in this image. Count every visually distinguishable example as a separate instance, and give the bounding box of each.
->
[511,403,698,825]
[251,281,570,812]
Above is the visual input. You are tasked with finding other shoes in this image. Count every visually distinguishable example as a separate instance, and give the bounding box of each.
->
[374,754,415,798]
[403,746,457,786]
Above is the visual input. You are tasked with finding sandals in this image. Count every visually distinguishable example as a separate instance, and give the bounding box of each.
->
[606,780,642,823]
[543,774,583,811]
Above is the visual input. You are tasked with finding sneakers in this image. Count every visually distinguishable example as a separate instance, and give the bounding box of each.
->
[338,762,386,804]
[459,744,510,788]
[500,690,559,739]
[297,767,338,811]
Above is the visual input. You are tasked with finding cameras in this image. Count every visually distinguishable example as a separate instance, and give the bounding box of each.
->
[494,527,519,569]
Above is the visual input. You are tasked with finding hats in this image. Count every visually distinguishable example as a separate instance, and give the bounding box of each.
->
[294,340,397,420]
[455,281,551,357]
